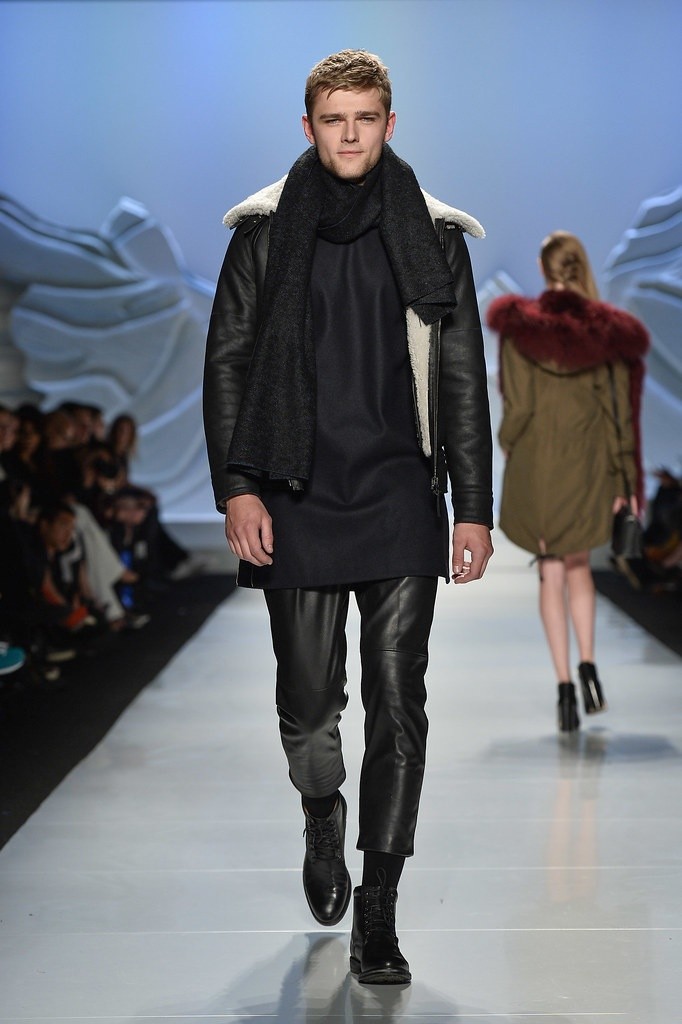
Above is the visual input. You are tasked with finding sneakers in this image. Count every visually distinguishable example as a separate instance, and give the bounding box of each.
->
[0,639,27,676]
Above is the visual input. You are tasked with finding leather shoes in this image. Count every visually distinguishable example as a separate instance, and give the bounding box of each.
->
[348,865,412,986]
[298,787,353,927]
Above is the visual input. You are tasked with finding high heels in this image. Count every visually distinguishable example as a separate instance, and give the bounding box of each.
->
[557,681,581,733]
[578,661,607,715]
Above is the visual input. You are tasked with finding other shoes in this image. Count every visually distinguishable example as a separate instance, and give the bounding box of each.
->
[44,641,79,665]
[111,611,150,635]
[121,569,142,585]
[39,657,62,682]
[170,550,206,582]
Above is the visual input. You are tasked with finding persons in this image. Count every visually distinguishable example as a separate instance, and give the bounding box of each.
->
[607,466,682,591]
[202,49,494,983]
[487,231,650,733]
[0,400,209,684]
